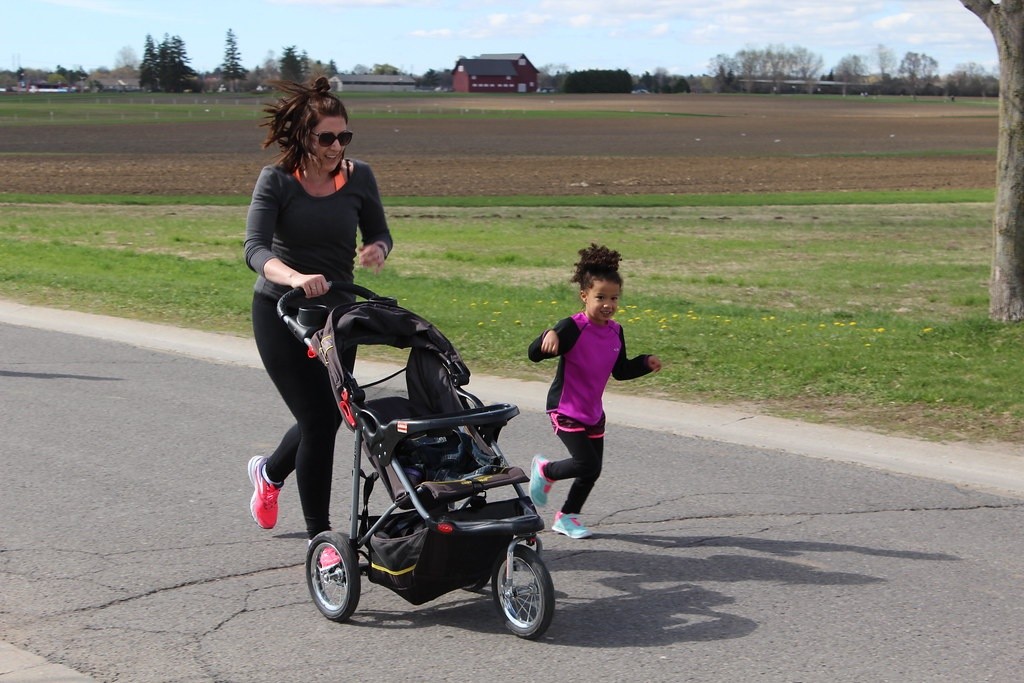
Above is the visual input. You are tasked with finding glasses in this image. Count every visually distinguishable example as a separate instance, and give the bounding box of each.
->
[311,130,354,147]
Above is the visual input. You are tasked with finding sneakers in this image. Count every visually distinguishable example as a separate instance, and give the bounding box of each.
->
[308,540,342,571]
[551,511,592,538]
[529,454,556,508]
[248,456,285,529]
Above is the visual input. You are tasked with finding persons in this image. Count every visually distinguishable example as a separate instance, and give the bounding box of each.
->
[528,244,663,541]
[244,74,394,573]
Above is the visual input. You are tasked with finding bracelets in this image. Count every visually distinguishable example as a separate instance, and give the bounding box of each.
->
[375,243,388,259]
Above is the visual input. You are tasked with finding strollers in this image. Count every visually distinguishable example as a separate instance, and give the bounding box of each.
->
[277,278,556,639]
